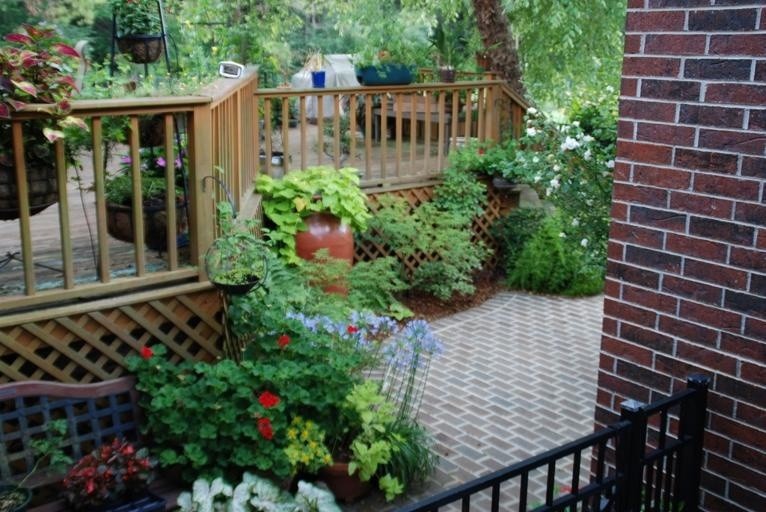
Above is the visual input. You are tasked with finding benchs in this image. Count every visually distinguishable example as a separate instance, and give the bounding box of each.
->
[0,372,213,507]
[369,104,477,155]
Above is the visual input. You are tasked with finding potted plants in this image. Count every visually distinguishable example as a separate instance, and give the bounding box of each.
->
[249,164,374,279]
[1,23,84,222]
[204,236,266,294]
[314,379,434,503]
[424,17,468,81]
[105,177,188,245]
[112,1,166,63]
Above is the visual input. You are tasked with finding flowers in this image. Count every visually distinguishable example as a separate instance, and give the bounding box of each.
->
[145,338,346,512]
